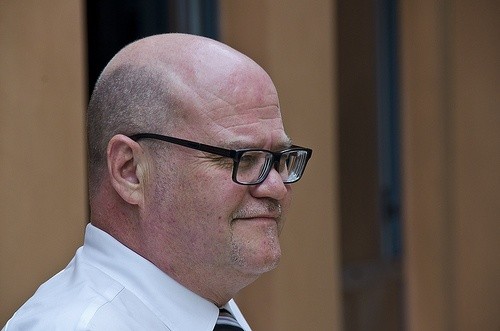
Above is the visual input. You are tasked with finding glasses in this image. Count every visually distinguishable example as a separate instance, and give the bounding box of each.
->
[128,133,312,186]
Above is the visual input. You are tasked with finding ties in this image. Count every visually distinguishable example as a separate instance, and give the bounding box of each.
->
[213,309,243,331]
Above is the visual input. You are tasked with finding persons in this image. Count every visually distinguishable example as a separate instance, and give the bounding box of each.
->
[0,33,313,331]
